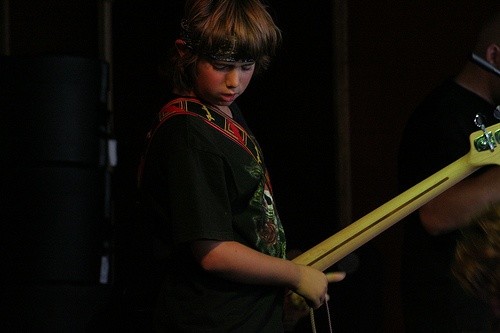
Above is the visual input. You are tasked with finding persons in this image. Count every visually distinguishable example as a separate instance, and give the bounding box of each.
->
[141,0,328,333]
[416,17,500,333]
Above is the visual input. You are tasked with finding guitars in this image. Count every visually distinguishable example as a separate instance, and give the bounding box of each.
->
[281,121,500,294]
